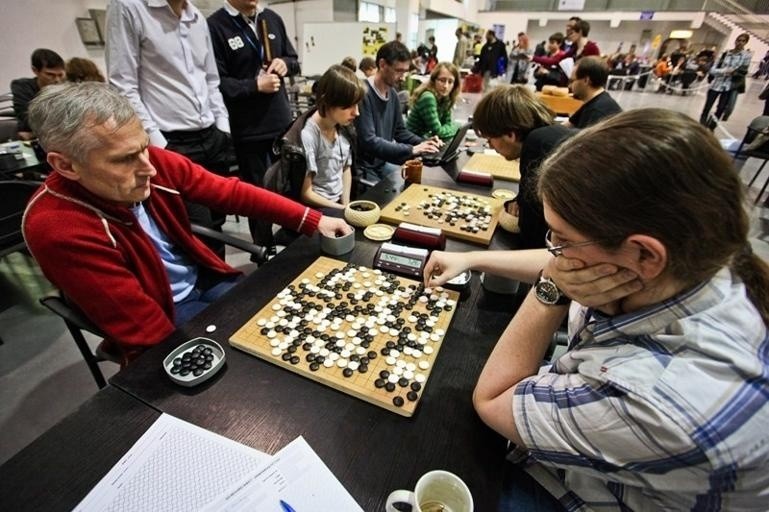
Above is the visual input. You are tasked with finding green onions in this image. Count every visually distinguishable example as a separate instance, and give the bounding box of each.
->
[380,184,507,246]
[228,255,460,417]
[462,153,523,183]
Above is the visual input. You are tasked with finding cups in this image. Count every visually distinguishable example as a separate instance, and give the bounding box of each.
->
[545,228,605,257]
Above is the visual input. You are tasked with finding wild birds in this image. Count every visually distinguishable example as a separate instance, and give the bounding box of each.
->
[413,122,472,166]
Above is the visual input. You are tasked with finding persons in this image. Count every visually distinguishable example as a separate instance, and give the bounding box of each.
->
[202,0,299,265]
[263,17,769,250]
[422,109,768,512]
[18,79,353,367]
[11,48,67,141]
[67,56,105,84]
[103,0,233,264]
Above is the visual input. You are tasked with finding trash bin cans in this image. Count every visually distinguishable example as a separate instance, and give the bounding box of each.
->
[532,268,572,306]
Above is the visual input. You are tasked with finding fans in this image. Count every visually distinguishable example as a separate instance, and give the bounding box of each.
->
[301,22,391,78]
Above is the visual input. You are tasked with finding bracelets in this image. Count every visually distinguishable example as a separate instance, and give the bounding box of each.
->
[343,200,381,228]
[317,224,357,257]
[497,207,520,233]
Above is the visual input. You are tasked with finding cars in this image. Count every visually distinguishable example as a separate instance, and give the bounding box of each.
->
[3,138,24,161]
[383,468,477,511]
[401,160,423,184]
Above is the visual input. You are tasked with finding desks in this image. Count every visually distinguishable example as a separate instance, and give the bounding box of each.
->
[1,385,420,512]
[109,208,531,512]
[352,72,584,300]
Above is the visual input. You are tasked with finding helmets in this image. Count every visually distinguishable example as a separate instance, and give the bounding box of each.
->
[161,336,226,389]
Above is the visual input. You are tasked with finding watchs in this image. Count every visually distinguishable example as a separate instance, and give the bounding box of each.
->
[532,268,572,306]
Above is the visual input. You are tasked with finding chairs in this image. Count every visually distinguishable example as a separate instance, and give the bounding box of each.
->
[730,115,769,207]
[456,55,769,97]
[2,92,55,312]
[39,215,266,388]
[224,71,369,223]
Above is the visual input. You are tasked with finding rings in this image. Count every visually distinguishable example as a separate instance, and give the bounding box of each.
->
[280,500,296,512]
